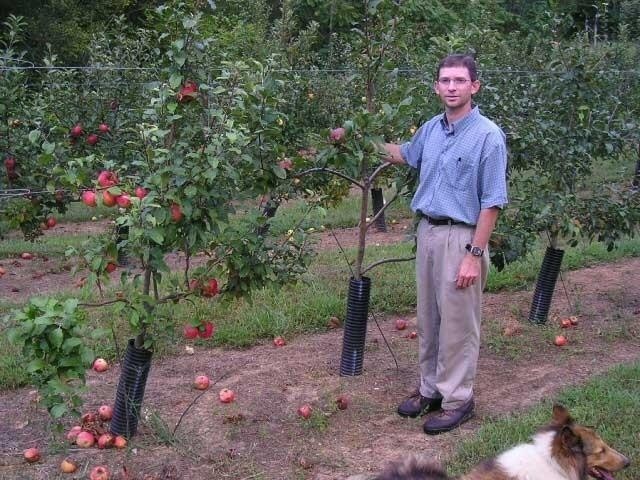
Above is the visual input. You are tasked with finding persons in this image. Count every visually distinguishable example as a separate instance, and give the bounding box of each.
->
[352,54,508,434]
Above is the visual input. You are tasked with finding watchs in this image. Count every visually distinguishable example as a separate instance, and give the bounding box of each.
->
[464,244,484,256]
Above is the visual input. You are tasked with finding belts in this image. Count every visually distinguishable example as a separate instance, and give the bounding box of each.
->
[421,212,463,226]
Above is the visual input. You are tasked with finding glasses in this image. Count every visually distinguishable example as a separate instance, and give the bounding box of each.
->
[437,76,473,84]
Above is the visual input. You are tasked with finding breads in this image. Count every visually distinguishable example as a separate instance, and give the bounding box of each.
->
[553,315,579,347]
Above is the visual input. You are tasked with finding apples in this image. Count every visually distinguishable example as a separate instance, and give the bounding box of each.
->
[0,80,418,480]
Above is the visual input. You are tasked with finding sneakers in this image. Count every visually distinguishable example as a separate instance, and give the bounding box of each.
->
[424,395,476,434]
[398,390,443,418]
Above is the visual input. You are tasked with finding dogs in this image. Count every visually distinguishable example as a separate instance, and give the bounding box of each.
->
[359,402,630,480]
